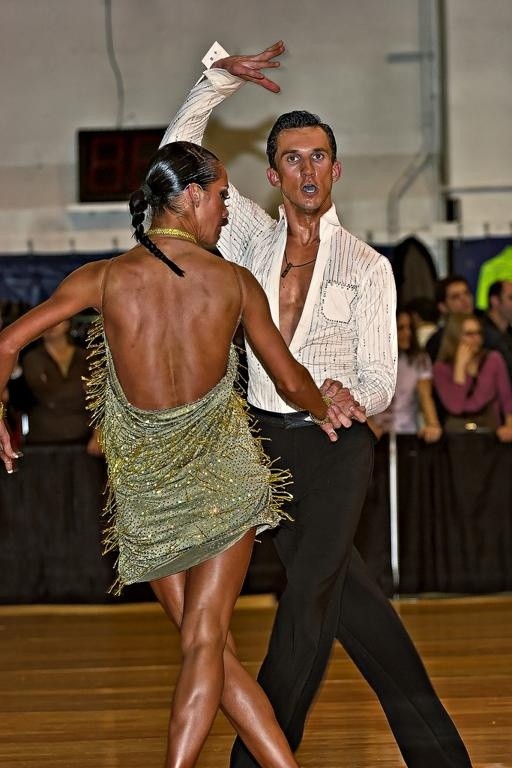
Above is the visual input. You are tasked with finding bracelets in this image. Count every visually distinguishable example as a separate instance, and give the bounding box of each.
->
[309,393,333,425]
[0,401,4,420]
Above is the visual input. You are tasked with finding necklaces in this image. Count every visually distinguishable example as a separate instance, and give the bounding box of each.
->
[281,249,316,278]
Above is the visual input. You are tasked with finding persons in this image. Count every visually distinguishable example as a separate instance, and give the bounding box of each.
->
[159,40,473,768]
[0,141,368,768]
[22,320,103,457]
[374,275,512,443]
